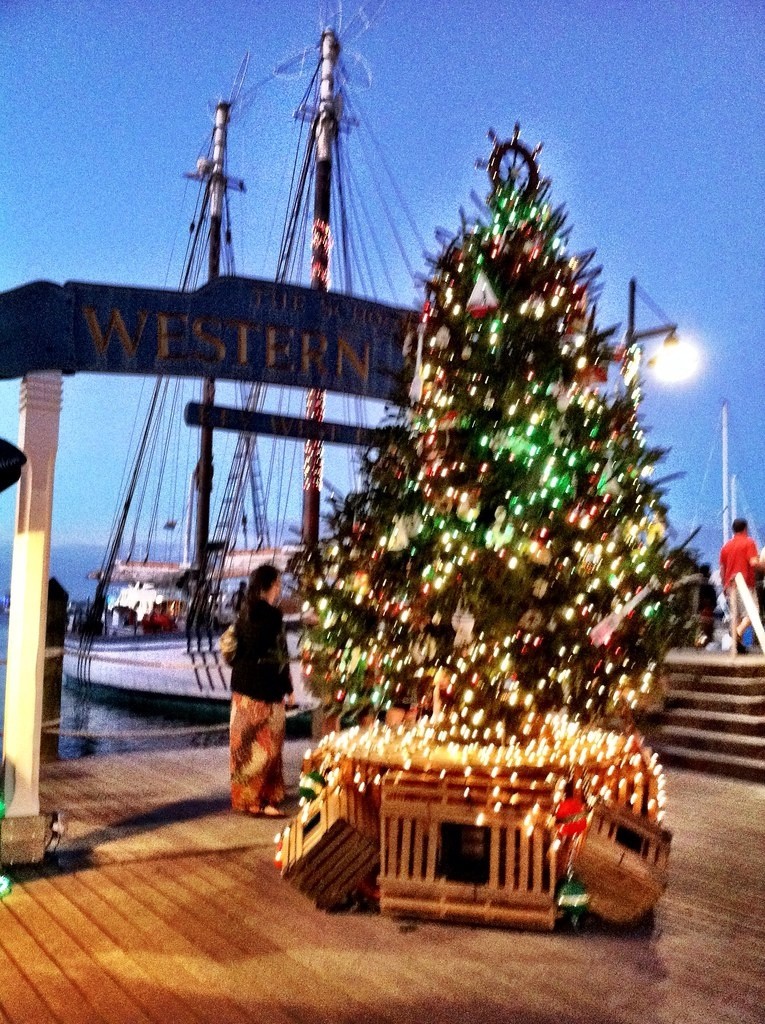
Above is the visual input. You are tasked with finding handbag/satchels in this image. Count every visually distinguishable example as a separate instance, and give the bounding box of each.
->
[219,624,238,665]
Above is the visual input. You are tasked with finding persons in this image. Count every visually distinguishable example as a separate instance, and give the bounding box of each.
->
[230,581,247,621]
[758,546,765,572]
[228,565,293,818]
[700,563,717,647]
[720,518,759,654]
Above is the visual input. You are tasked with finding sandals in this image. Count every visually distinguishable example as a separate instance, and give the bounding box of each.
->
[245,803,286,819]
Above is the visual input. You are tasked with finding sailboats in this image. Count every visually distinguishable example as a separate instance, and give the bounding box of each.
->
[54,25,341,712]
